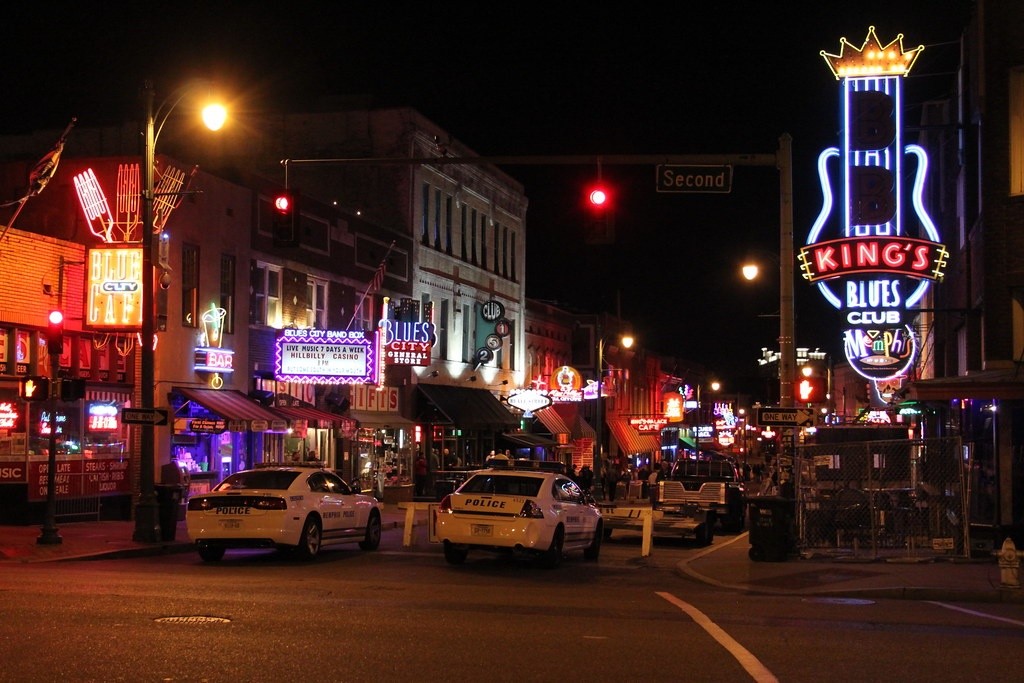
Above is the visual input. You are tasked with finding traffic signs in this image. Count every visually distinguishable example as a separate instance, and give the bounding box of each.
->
[121,408,169,426]
[756,407,814,427]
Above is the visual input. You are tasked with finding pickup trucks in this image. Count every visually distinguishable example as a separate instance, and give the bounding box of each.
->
[657,458,746,533]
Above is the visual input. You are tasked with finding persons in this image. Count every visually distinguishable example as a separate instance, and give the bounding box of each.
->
[199,456,208,472]
[416,448,462,495]
[567,461,672,502]
[733,444,791,495]
[485,448,514,462]
[818,481,871,548]
[307,451,319,460]
[291,452,301,461]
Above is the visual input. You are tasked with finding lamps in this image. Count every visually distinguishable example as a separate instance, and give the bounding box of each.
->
[487,380,508,386]
[419,371,439,380]
[453,376,477,384]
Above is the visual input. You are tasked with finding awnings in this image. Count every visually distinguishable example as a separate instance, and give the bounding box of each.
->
[266,405,358,431]
[534,406,571,440]
[679,438,700,450]
[414,383,521,429]
[607,419,662,456]
[172,387,287,432]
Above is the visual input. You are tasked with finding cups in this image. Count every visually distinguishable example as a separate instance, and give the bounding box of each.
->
[199,462,208,472]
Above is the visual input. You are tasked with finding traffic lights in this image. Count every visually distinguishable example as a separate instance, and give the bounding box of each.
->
[48,309,64,353]
[793,377,824,402]
[271,190,299,247]
[22,375,49,401]
[586,182,616,247]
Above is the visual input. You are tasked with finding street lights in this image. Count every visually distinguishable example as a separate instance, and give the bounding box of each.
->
[592,329,636,483]
[695,375,722,459]
[131,78,242,542]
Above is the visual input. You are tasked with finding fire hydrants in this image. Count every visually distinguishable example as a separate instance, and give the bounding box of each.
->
[990,536,1024,590]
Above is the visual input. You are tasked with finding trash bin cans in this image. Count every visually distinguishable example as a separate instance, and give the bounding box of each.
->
[747,497,792,563]
[706,485,748,536]
[629,480,642,498]
[154,483,183,541]
[177,483,190,521]
[434,479,457,504]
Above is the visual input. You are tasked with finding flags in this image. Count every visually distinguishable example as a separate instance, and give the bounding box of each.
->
[369,258,387,291]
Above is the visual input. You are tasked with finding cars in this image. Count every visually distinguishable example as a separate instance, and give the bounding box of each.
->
[434,459,603,570]
[185,462,383,563]
